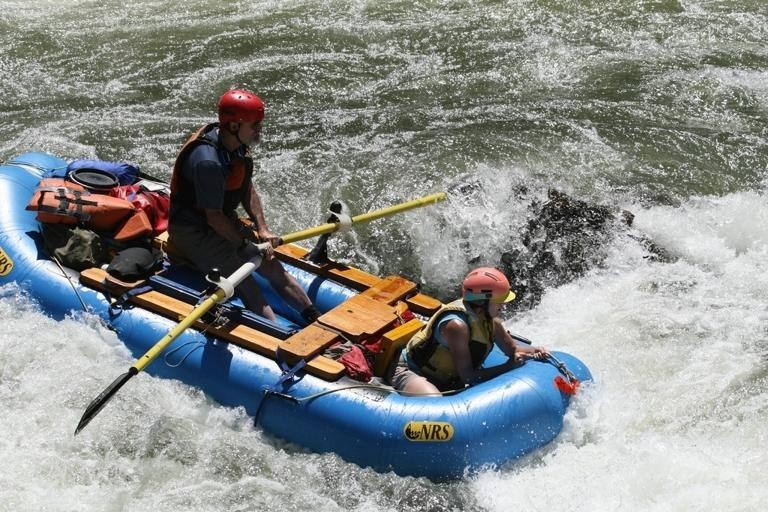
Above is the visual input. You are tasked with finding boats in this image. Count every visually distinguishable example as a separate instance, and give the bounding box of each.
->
[1,150,593,486]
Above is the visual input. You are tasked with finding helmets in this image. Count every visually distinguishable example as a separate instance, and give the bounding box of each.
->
[460,266,517,304]
[217,88,266,133]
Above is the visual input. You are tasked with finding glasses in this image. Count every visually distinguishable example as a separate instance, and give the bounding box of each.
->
[230,119,263,132]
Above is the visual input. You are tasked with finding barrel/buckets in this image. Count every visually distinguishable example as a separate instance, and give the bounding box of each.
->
[69,168,120,194]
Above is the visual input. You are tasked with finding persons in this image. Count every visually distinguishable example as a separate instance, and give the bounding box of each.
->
[386,267,545,397]
[167,89,321,329]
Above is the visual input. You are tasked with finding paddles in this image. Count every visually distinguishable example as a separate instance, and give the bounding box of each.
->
[271,179,485,247]
[74,253,265,437]
